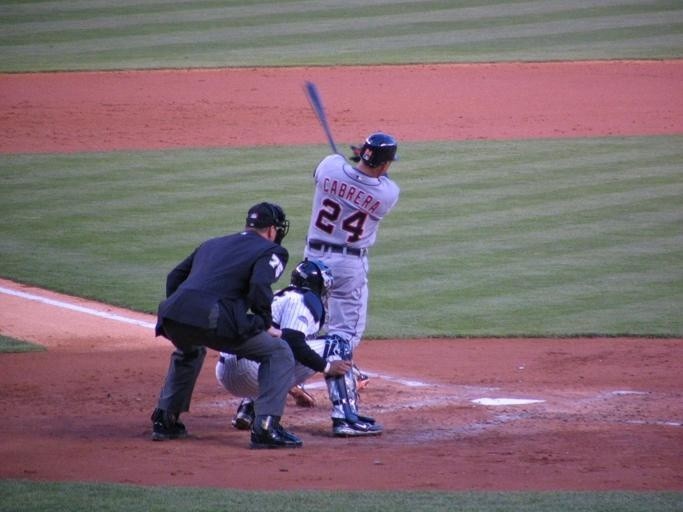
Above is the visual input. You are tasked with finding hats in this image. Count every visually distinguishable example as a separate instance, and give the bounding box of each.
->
[246,201,291,247]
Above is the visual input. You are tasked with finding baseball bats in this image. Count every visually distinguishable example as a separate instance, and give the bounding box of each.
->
[305,81,336,154]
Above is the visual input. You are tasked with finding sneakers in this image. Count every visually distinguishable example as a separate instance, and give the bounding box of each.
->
[332,415,384,436]
[231,398,256,432]
[353,372,371,392]
[150,416,189,441]
[287,382,317,408]
[250,425,303,449]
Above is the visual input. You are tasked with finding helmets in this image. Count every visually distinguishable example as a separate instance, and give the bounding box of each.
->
[360,132,399,168]
[291,258,334,300]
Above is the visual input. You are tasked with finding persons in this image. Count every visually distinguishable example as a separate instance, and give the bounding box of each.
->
[217,261,382,439]
[252,135,400,405]
[149,204,302,452]
